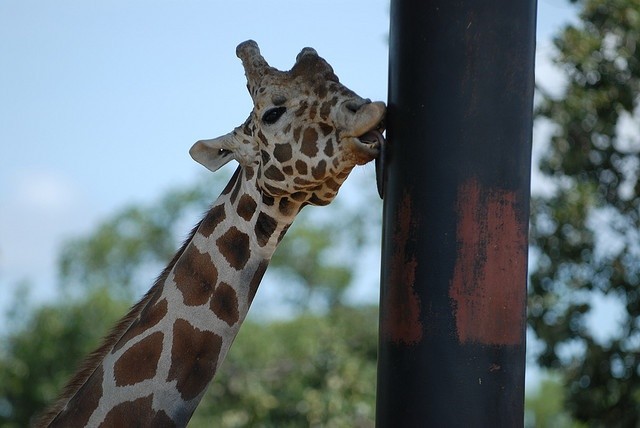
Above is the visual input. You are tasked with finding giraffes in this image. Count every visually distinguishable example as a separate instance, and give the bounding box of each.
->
[28,39,387,428]
